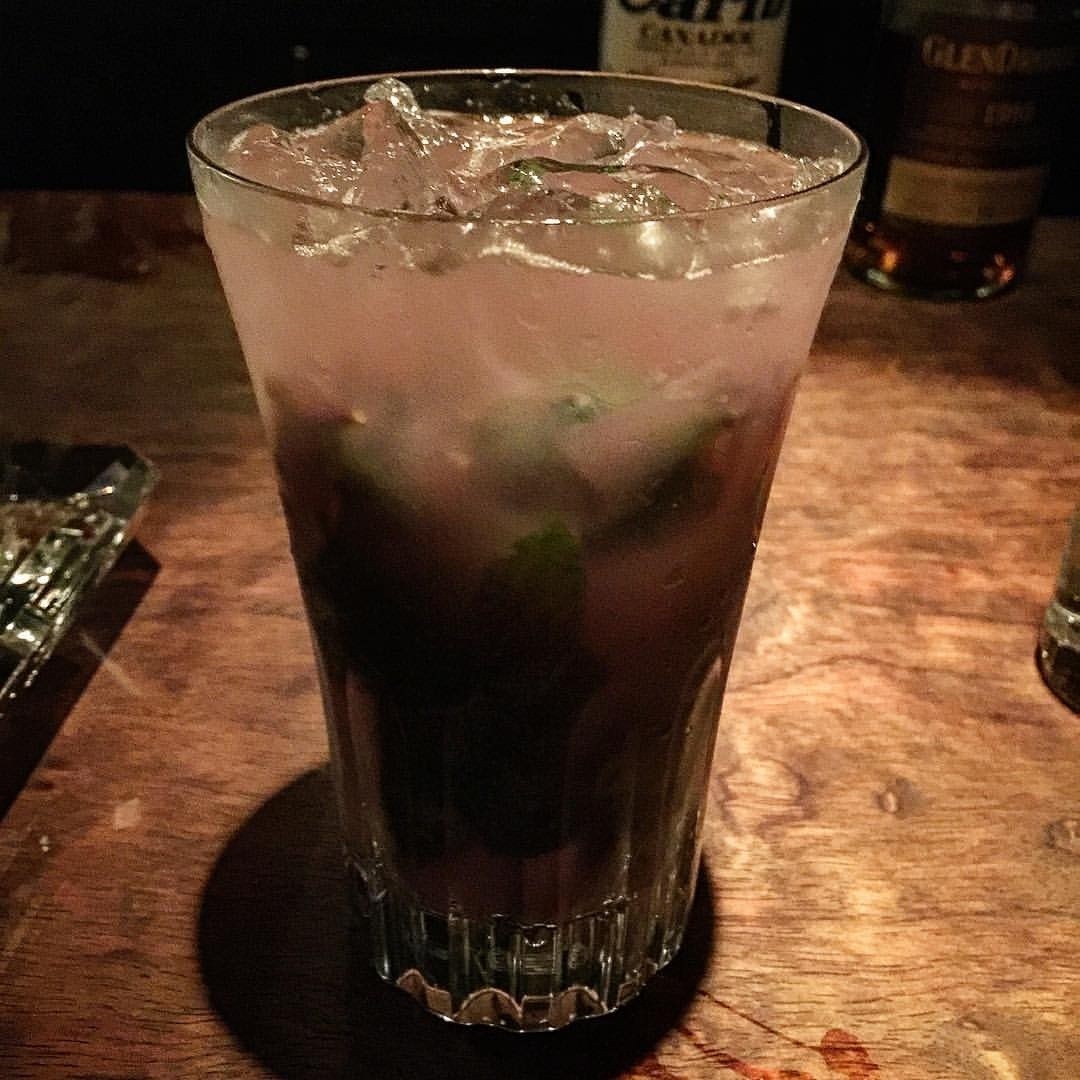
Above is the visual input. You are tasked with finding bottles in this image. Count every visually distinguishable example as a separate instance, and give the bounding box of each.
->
[1034,486,1080,717]
[587,1,793,99]
[837,1,1058,307]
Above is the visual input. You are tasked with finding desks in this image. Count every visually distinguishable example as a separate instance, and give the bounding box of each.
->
[0,185,1077,1080]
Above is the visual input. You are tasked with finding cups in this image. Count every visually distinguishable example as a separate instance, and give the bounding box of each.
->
[183,63,870,1036]
